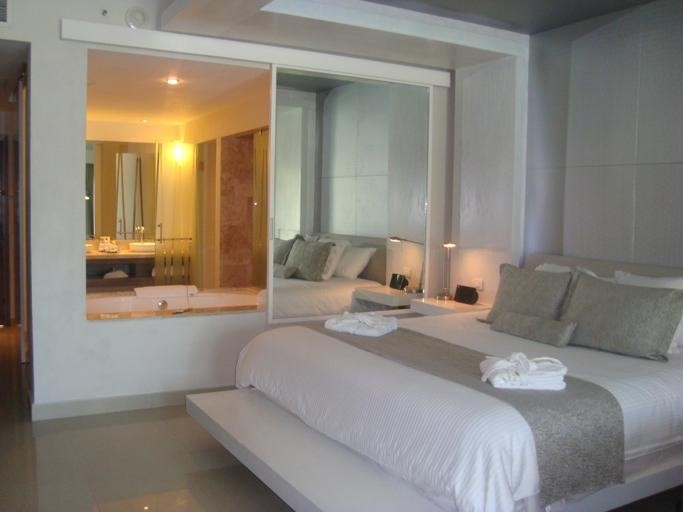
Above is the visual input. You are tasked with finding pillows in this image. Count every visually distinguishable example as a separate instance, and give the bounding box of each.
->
[273,263,296,278]
[274,237,295,265]
[285,239,333,281]
[614,269,683,354]
[318,238,349,279]
[563,274,683,362]
[336,246,376,279]
[535,263,615,285]
[486,263,572,327]
[491,312,574,347]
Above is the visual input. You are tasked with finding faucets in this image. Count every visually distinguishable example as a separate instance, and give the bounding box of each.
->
[135,226,146,241]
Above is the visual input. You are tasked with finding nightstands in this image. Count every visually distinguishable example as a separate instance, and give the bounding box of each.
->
[354,285,421,308]
[411,297,490,317]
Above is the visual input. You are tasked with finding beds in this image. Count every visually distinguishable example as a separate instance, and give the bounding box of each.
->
[273,231,386,317]
[185,254,683,512]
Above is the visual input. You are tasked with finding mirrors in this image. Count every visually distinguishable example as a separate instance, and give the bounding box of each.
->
[86,140,160,241]
[270,66,431,319]
[85,48,269,320]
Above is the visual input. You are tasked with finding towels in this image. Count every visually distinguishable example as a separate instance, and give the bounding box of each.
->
[478,351,567,391]
[324,310,398,337]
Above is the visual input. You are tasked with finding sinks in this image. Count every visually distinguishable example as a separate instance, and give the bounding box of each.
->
[129,242,156,251]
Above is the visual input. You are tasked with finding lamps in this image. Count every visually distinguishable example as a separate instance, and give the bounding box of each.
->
[388,237,426,292]
[442,239,457,296]
